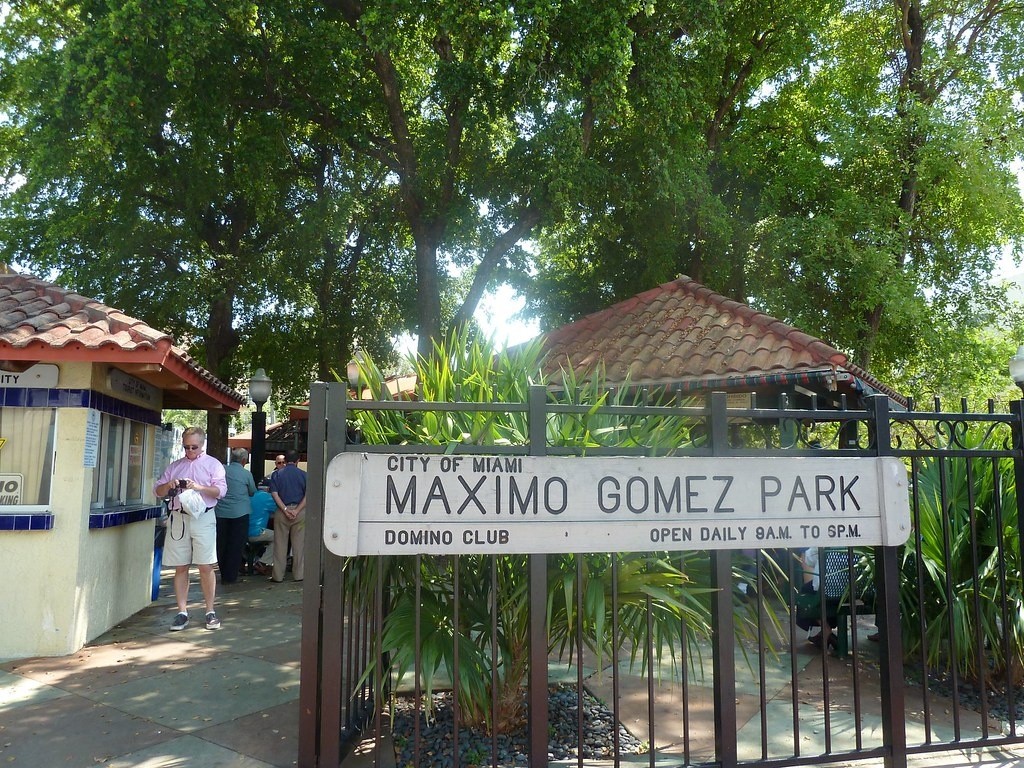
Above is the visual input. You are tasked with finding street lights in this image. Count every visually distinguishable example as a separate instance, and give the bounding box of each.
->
[247,368,274,490]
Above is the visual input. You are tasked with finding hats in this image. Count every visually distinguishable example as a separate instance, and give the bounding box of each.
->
[257,479,272,489]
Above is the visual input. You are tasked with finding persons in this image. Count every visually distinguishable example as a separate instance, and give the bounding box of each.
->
[214,448,257,585]
[269,451,307,583]
[267,455,286,479]
[248,480,277,572]
[795,545,873,656]
[152,427,228,630]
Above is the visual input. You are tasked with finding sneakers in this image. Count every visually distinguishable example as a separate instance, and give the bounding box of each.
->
[169,614,189,630]
[205,612,220,629]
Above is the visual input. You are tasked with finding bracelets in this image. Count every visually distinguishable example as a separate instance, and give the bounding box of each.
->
[283,507,288,513]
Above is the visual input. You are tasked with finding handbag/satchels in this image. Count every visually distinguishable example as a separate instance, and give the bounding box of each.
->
[178,489,207,520]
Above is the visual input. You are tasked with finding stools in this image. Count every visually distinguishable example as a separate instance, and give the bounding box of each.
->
[241,541,269,576]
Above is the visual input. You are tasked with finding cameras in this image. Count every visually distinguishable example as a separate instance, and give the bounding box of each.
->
[176,480,187,489]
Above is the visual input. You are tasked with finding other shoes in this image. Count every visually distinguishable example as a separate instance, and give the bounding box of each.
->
[867,633,880,642]
[253,561,269,575]
[808,632,831,650]
[267,577,282,583]
[294,579,303,582]
[829,634,839,651]
[221,577,243,585]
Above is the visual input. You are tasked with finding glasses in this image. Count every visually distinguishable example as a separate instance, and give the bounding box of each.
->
[277,461,285,465]
[183,444,198,450]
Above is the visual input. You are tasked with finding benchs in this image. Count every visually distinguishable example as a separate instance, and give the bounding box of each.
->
[836,599,876,659]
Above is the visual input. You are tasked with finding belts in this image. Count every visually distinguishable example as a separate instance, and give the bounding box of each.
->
[285,503,299,506]
[175,507,213,514]
